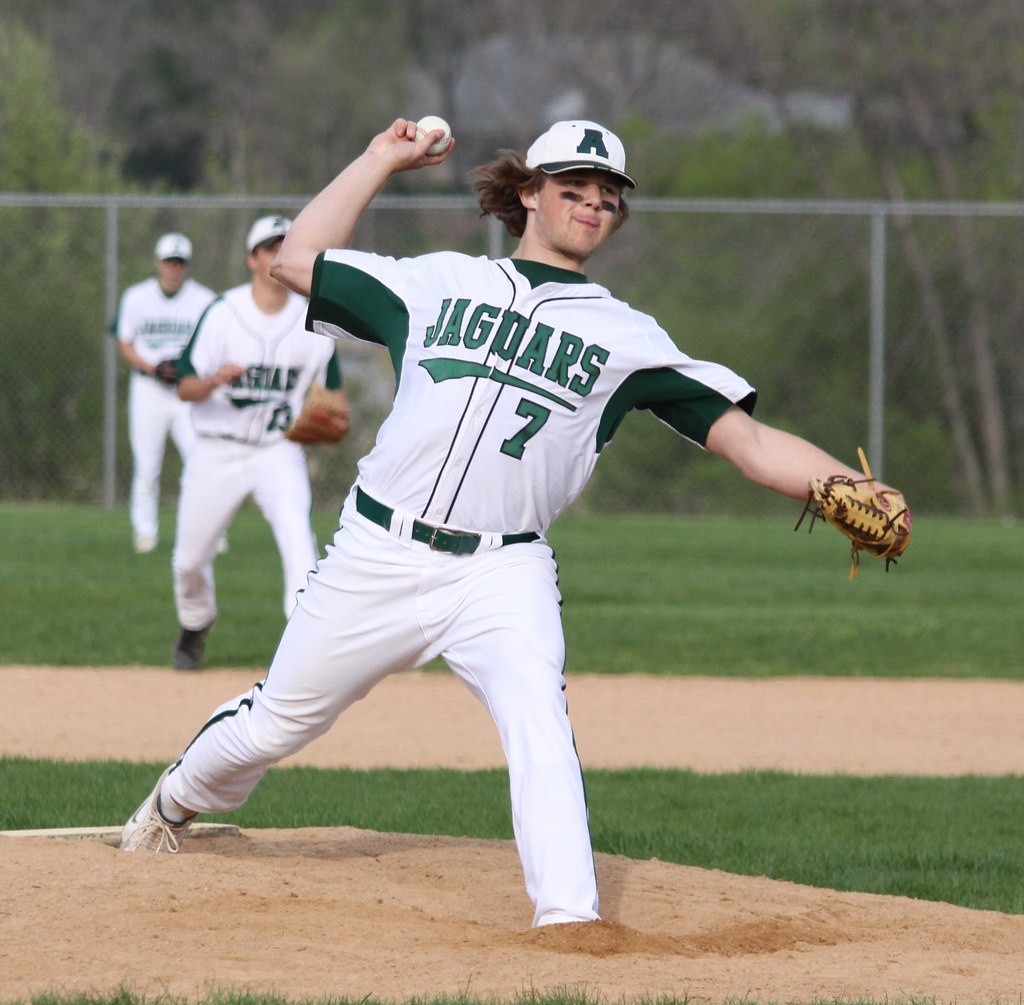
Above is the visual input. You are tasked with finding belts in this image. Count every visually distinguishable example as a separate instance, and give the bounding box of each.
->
[352,486,543,554]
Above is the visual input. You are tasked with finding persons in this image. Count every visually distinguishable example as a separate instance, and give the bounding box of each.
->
[108,229,219,552]
[171,212,351,673]
[119,119,907,929]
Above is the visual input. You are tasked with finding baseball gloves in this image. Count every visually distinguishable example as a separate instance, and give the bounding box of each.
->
[156,360,179,386]
[808,477,912,559]
[284,384,352,446]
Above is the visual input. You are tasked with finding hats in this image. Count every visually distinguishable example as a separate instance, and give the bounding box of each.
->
[246,214,294,254]
[525,119,637,187]
[155,234,193,261]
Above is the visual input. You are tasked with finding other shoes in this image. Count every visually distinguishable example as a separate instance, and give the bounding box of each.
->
[174,618,213,668]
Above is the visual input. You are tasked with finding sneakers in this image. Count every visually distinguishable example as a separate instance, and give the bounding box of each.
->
[118,760,197,855]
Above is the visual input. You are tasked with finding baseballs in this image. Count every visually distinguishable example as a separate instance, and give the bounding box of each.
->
[415,114,452,156]
